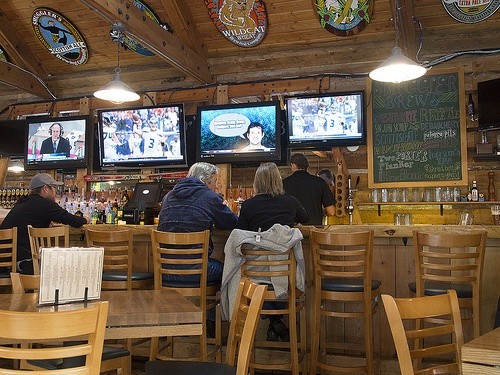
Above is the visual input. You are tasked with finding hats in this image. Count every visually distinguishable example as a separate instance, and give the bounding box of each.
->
[29,173,64,189]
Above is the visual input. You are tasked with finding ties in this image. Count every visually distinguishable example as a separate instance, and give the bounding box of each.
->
[52,140,57,153]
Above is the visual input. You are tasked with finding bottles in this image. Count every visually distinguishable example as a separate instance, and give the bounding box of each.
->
[137,211,145,226]
[466,180,479,202]
[0,185,129,225]
[219,188,245,217]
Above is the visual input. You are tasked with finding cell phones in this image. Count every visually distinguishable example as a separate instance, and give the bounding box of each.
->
[315,224,323,227]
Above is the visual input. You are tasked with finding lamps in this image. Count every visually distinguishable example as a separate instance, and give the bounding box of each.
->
[369,0,432,81]
[93,22,140,105]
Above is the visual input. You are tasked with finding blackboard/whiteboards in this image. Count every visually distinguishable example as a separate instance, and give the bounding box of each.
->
[365,66,469,189]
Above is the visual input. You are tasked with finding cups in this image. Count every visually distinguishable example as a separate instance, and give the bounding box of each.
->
[394,213,412,225]
[491,205,499,225]
[461,213,474,226]
[370,187,462,202]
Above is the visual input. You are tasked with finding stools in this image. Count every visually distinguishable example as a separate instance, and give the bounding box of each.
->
[0,223,488,375]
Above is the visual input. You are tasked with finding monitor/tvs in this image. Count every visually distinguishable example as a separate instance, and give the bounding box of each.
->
[285,92,365,148]
[23,114,89,169]
[96,103,188,170]
[197,100,282,162]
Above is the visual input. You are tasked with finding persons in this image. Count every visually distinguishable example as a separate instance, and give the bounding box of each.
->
[291,96,358,135]
[156,162,238,338]
[0,173,91,293]
[318,169,333,186]
[283,154,335,225]
[242,122,266,148]
[102,107,181,160]
[40,123,71,157]
[236,162,308,341]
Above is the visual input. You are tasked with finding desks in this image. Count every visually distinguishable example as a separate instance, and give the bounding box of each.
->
[459,326,500,375]
[0,289,204,339]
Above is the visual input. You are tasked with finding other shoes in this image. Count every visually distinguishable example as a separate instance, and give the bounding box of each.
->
[204,319,216,338]
[266,319,291,342]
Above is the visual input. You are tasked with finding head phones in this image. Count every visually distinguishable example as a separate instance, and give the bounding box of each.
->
[48,122,64,134]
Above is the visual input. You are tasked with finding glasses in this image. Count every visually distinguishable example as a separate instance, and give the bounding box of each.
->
[46,185,60,192]
